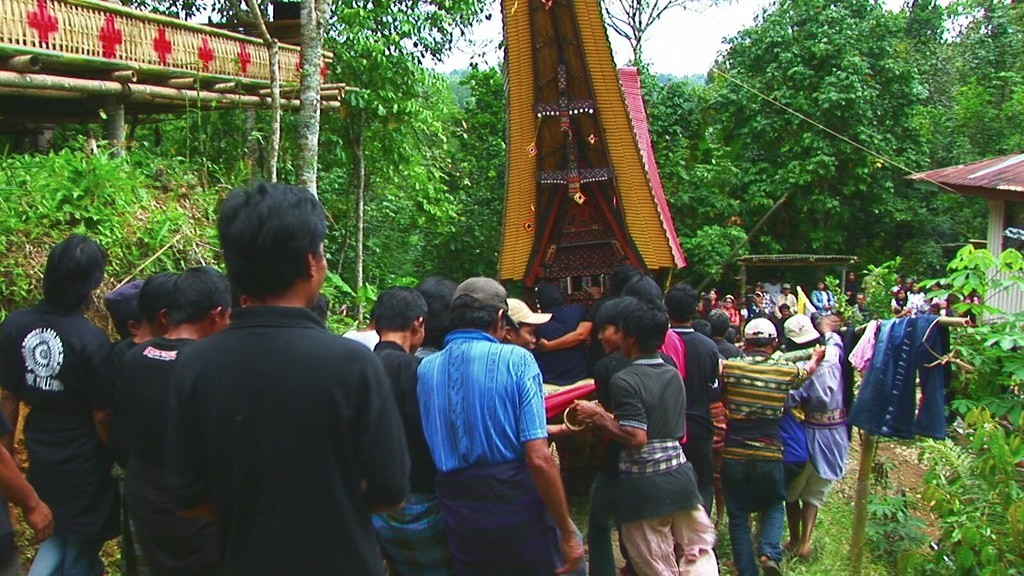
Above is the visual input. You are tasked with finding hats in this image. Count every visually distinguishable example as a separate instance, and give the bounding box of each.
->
[722,295,736,308]
[103,280,145,320]
[744,318,777,339]
[451,278,519,331]
[755,291,763,298]
[506,298,553,327]
[782,283,791,289]
[783,314,820,344]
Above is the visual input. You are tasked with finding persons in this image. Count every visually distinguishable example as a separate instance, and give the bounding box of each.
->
[0,181,981,576]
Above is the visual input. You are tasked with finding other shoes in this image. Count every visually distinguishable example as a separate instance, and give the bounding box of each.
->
[766,561,781,576]
